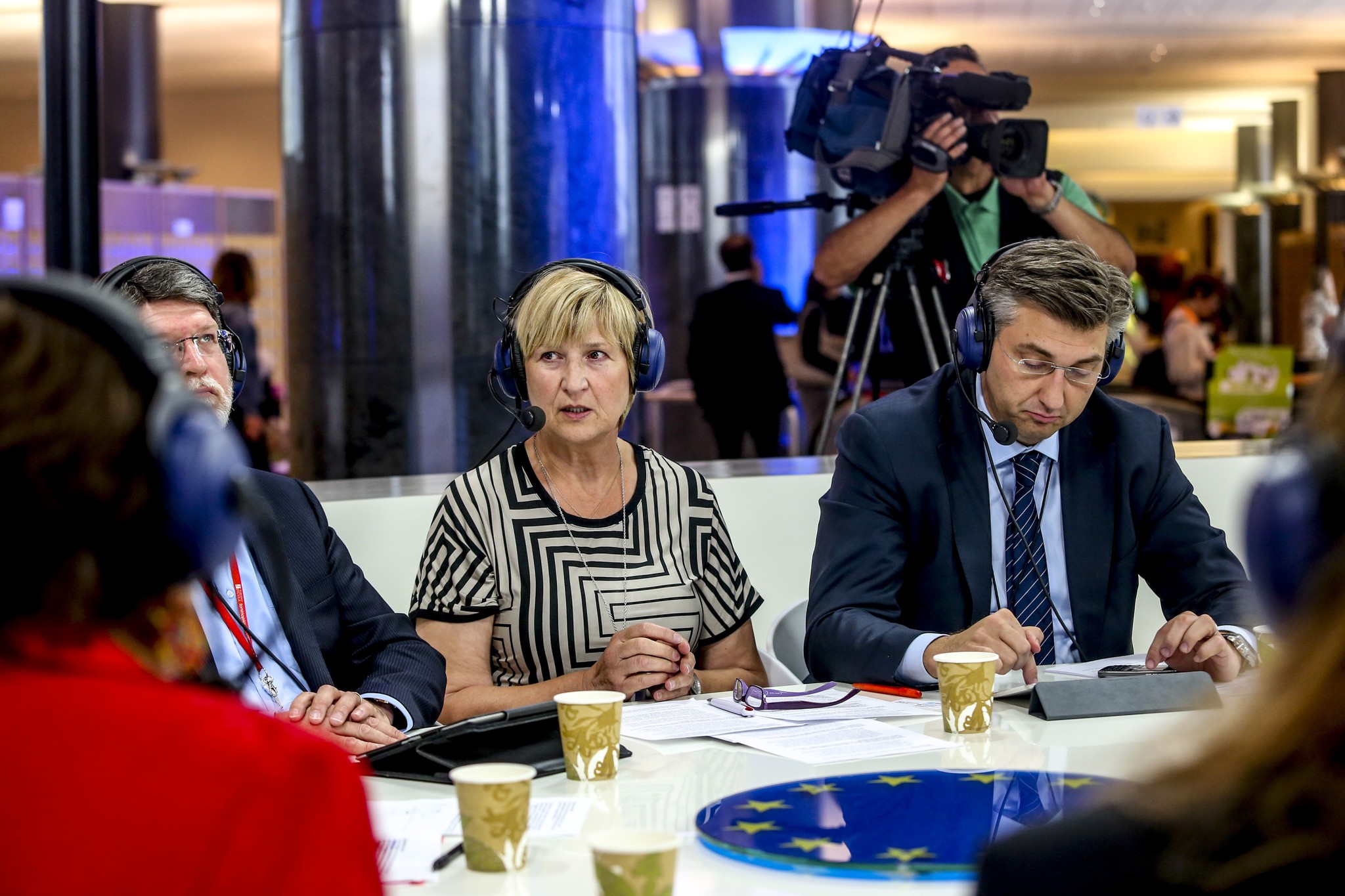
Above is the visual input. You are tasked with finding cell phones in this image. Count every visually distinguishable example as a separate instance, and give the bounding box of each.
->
[1098,663,1177,677]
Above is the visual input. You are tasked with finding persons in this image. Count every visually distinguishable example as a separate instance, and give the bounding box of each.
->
[811,43,1138,387]
[210,247,273,474]
[87,255,447,755]
[802,237,1274,685]
[407,258,771,726]
[0,265,379,896]
[682,230,798,464]
[805,233,1343,432]
[974,320,1345,896]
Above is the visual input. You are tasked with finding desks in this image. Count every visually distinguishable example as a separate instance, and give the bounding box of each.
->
[342,665,1243,895]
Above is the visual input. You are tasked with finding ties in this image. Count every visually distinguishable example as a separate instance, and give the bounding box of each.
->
[1006,452,1057,665]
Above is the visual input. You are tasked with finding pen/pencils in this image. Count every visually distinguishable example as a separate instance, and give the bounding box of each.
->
[707,697,755,717]
[431,840,462,869]
[851,682,923,699]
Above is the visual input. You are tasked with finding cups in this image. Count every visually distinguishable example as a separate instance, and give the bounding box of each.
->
[588,839,677,896]
[449,762,538,871]
[933,651,999,734]
[553,691,626,780]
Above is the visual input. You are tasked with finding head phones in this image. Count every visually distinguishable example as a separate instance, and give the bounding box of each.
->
[92,255,248,384]
[489,257,664,433]
[950,237,1128,446]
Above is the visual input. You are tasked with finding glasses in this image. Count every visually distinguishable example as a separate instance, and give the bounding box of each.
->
[994,327,1110,386]
[156,328,235,363]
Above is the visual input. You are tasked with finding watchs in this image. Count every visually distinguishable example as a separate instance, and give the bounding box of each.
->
[373,698,397,720]
[1028,178,1066,216]
[1218,631,1258,674]
[688,672,703,697]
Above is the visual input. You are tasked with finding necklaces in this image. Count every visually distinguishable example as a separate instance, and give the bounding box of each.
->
[531,431,628,635]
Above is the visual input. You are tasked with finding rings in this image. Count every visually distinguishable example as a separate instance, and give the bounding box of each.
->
[352,691,363,705]
[1206,623,1218,639]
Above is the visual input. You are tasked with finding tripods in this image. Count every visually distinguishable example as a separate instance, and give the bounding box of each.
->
[713,182,954,457]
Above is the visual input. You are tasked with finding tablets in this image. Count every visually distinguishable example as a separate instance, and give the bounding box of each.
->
[356,689,632,788]
